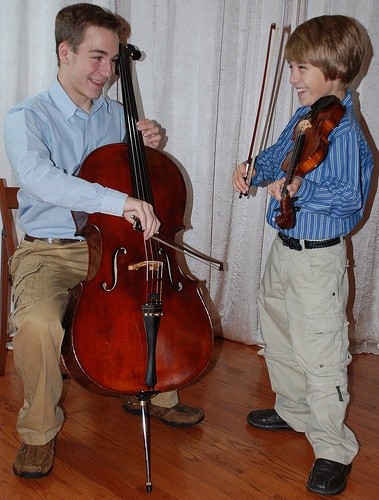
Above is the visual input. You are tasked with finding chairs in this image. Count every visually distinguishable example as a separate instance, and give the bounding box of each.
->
[0,178,68,378]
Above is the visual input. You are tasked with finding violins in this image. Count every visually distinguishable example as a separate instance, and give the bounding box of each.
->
[272,94,347,231]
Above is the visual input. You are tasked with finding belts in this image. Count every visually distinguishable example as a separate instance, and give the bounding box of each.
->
[24,234,78,246]
[278,231,346,252]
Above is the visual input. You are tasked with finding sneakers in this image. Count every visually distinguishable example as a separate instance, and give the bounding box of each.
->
[124,395,205,427]
[13,436,56,478]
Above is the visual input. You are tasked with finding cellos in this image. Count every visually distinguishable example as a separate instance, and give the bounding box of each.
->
[61,41,214,397]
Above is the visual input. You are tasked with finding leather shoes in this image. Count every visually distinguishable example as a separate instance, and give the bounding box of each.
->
[307,459,351,495]
[247,410,289,429]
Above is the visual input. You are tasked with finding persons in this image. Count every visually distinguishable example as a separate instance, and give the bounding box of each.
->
[232,15,374,496]
[3,3,206,478]
